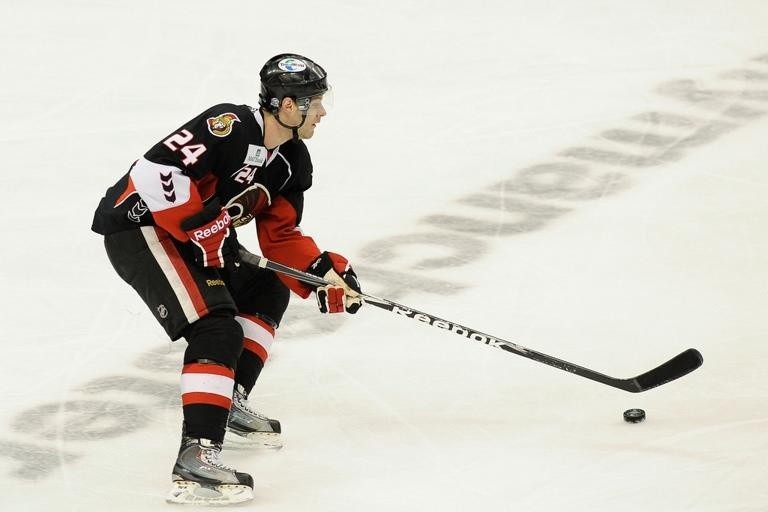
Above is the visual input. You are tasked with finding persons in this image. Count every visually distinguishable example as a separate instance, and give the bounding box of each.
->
[91,53,361,489]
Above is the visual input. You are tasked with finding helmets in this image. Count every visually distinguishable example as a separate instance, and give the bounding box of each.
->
[260,54,334,115]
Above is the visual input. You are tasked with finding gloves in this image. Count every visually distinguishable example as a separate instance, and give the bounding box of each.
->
[179,196,258,279]
[299,251,364,313]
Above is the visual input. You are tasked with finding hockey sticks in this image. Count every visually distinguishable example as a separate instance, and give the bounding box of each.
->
[240,250,703,393]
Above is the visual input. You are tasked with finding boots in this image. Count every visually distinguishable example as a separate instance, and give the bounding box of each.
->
[227,383,281,435]
[173,438,252,490]
